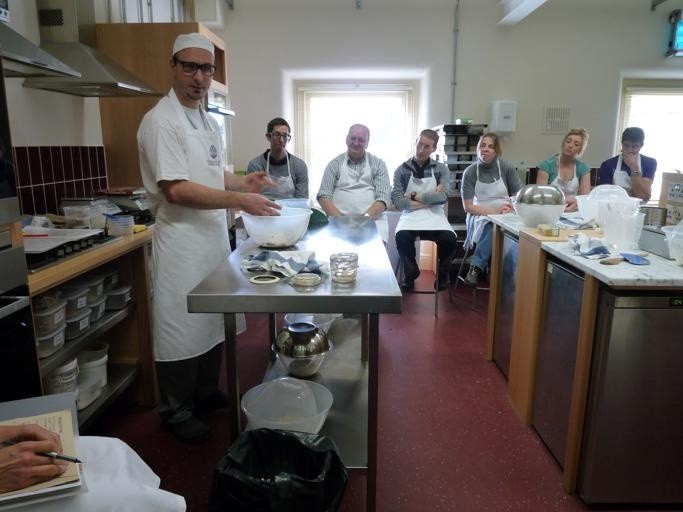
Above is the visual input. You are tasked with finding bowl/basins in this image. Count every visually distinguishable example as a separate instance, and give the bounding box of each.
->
[240,377,335,436]
[513,184,683,269]
[238,204,315,252]
[266,310,336,376]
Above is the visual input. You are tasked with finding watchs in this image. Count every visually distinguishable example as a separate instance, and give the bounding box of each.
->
[410,190,416,202]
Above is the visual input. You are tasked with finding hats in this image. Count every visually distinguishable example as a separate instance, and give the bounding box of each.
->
[173,33,215,57]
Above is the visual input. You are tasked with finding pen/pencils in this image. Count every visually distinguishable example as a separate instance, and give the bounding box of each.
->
[2,441,83,463]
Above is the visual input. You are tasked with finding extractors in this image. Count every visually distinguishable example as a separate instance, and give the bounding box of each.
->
[0,0,81,96]
[32,0,164,107]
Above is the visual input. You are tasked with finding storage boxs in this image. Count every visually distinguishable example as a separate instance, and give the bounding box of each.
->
[33,268,132,358]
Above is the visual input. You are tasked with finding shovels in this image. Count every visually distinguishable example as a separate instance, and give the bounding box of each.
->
[600,253,650,265]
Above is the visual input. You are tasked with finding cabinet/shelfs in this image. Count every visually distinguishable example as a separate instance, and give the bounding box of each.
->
[431,124,488,199]
[38,303,138,431]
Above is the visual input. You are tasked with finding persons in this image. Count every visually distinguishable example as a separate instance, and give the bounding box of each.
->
[1,423,68,495]
[599,129,658,202]
[247,117,308,202]
[316,125,392,251]
[134,33,283,434]
[391,128,457,292]
[460,133,526,285]
[535,127,591,215]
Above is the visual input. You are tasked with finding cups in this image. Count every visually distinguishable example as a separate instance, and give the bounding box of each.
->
[330,252,358,284]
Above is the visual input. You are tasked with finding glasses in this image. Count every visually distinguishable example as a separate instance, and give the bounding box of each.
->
[176,58,216,76]
[270,132,288,140]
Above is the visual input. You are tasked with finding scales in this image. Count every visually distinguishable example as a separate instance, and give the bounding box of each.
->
[59,186,151,215]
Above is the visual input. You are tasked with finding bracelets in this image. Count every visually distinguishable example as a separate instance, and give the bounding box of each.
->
[630,170,641,176]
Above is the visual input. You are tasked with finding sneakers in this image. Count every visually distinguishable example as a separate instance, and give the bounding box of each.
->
[160,417,214,442]
[435,277,447,290]
[400,270,420,291]
[465,265,481,286]
[195,390,242,413]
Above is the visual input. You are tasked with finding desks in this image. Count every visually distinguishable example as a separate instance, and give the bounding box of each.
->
[184,213,404,511]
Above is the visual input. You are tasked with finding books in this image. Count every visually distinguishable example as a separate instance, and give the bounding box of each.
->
[0,391,91,512]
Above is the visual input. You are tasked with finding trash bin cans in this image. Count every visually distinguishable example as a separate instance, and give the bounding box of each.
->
[215,427,334,512]
